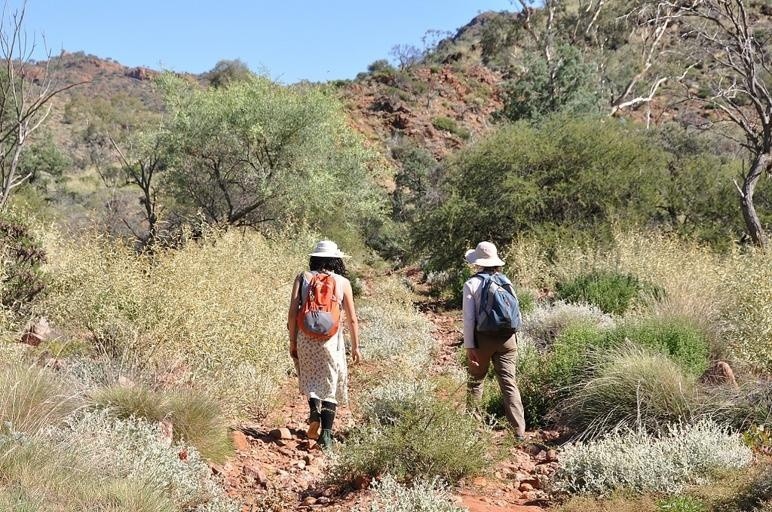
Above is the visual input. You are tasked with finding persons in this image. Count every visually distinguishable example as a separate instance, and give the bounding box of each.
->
[461,241,528,445]
[287,240,360,445]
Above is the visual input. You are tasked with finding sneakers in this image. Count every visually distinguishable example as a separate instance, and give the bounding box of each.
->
[319,429,332,449]
[307,412,320,441]
[501,434,523,442]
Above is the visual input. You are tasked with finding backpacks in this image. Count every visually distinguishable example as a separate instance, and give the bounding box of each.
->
[471,272,519,336]
[297,271,340,339]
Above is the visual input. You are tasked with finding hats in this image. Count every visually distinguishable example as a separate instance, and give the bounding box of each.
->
[307,240,352,258]
[465,241,505,268]
